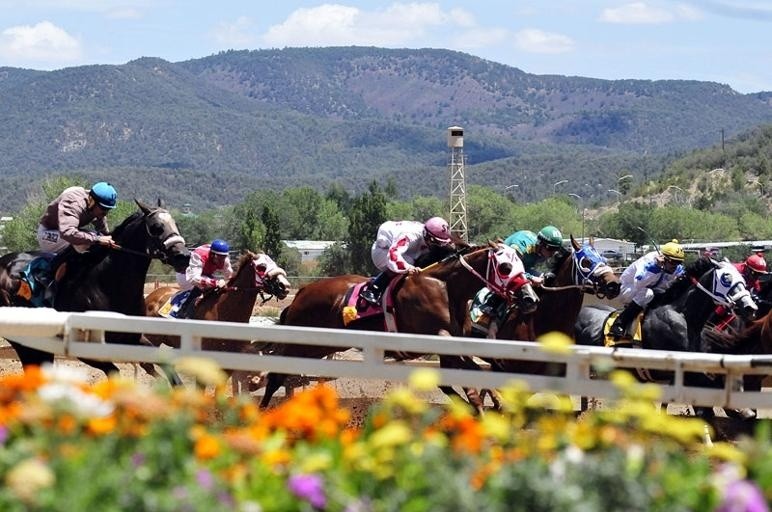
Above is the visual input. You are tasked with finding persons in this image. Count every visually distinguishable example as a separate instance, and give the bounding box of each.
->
[703,251,769,334]
[359,216,454,308]
[175,238,236,319]
[607,241,688,338]
[34,181,118,295]
[468,224,563,324]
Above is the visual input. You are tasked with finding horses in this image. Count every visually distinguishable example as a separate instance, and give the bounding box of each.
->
[140,247,291,397]
[746,272,772,319]
[467,233,624,415]
[0,195,192,383]
[573,252,759,443]
[240,237,541,419]
[702,309,772,426]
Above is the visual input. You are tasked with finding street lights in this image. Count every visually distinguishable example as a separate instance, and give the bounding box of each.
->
[606,186,624,208]
[568,192,586,244]
[706,167,723,194]
[631,223,662,256]
[667,182,684,203]
[616,174,635,206]
[747,180,765,200]
[503,183,520,199]
[552,177,569,196]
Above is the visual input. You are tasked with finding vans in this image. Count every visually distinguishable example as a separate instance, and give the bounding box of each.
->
[0,216,14,232]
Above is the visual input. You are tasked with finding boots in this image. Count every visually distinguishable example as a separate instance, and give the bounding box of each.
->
[610,300,642,336]
[35,243,81,287]
[176,286,201,318]
[360,266,397,303]
[480,294,502,314]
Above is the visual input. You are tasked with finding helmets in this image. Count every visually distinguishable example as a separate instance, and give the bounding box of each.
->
[90,182,117,210]
[660,239,685,263]
[537,226,564,248]
[746,252,768,275]
[424,215,452,243]
[210,240,230,256]
